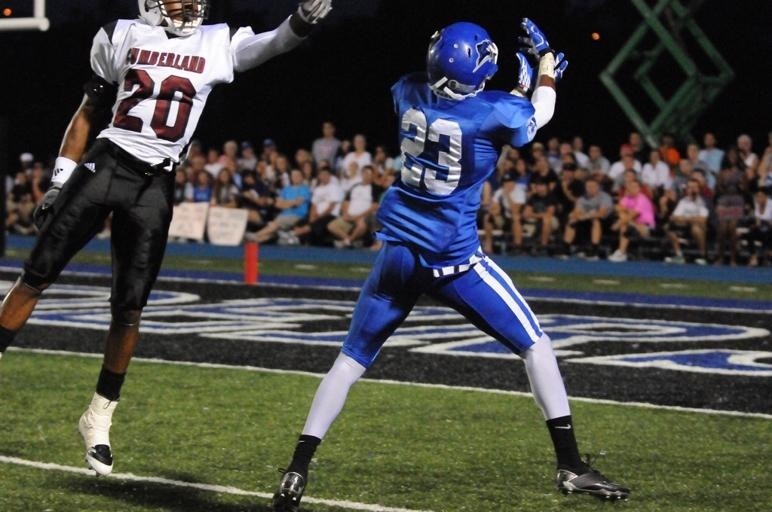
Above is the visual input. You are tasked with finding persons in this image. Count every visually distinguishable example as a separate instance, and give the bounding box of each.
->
[479,133,772,269]
[174,121,401,249]
[1,1,334,479]
[6,152,113,240]
[268,17,631,506]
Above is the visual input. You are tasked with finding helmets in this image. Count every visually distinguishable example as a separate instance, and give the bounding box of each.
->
[137,0,211,36]
[427,22,500,93]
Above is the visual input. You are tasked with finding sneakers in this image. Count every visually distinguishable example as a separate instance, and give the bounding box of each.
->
[663,255,685,264]
[576,249,599,261]
[271,468,307,510]
[608,250,629,262]
[547,247,571,261]
[555,462,631,501]
[78,405,114,475]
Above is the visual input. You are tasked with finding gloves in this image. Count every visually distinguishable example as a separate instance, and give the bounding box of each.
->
[518,18,556,61]
[515,49,570,97]
[296,0,334,27]
[32,183,64,230]
